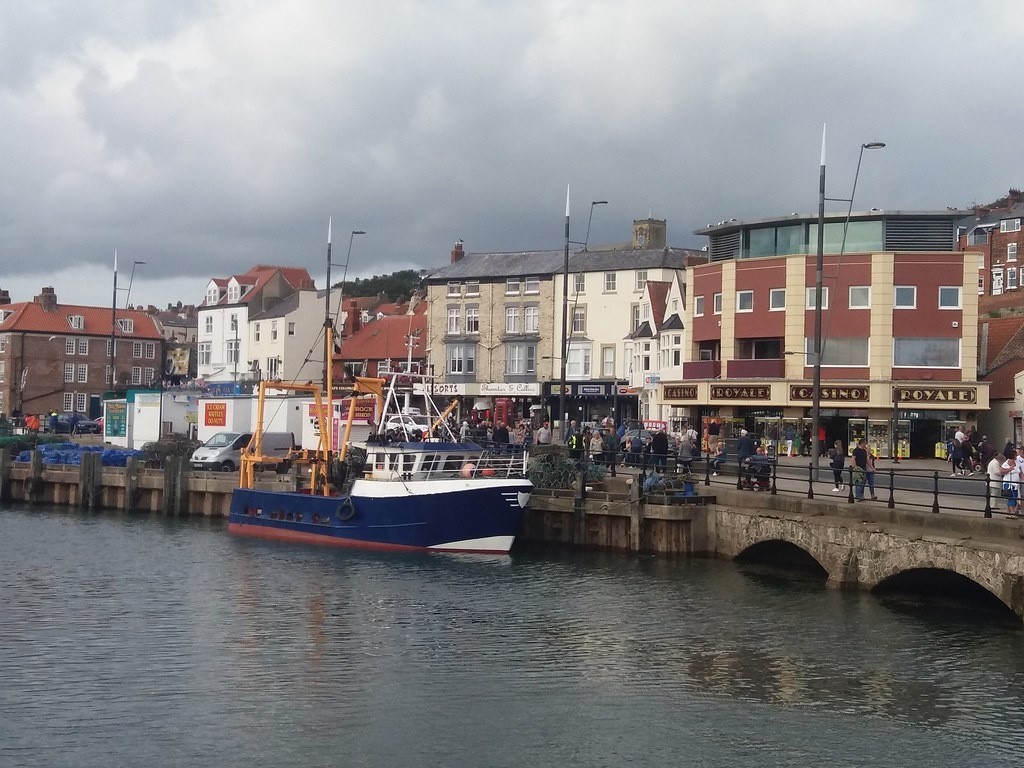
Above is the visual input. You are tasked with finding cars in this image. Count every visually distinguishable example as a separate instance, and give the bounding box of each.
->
[578,421,610,438]
[93,417,104,433]
[385,416,444,438]
[618,429,678,455]
[45,411,99,434]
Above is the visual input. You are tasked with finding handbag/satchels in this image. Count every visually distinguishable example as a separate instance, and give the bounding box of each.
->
[1001,472,1012,497]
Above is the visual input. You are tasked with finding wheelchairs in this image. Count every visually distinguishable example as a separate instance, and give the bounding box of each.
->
[968,449,983,472]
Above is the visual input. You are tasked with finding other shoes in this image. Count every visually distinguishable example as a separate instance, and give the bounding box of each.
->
[857,494,877,502]
[712,471,721,478]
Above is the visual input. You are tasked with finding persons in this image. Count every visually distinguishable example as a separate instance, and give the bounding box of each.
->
[947,438,966,476]
[794,435,801,457]
[861,445,878,501]
[686,425,699,443]
[954,425,1012,474]
[960,436,974,477]
[677,428,772,490]
[1015,446,1024,514]
[801,426,812,456]
[459,420,551,446]
[621,436,633,467]
[639,437,652,470]
[987,454,1014,508]
[12,407,80,436]
[1000,449,1021,519]
[563,420,621,477]
[830,440,845,492]
[852,439,877,501]
[818,426,828,459]
[651,429,669,473]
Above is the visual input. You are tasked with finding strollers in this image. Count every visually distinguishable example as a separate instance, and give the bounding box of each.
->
[741,455,771,491]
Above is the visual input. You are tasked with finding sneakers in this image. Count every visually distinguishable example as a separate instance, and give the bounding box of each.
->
[949,469,963,477]
[963,469,975,477]
[831,484,845,492]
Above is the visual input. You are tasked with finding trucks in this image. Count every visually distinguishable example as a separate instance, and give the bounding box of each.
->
[189,431,296,474]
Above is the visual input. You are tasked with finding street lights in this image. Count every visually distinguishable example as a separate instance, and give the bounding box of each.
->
[541,184,608,441]
[781,122,887,484]
[231,319,238,382]
[322,216,366,397]
[109,249,148,390]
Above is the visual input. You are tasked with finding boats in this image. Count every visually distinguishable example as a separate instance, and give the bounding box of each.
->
[226,441,533,555]
